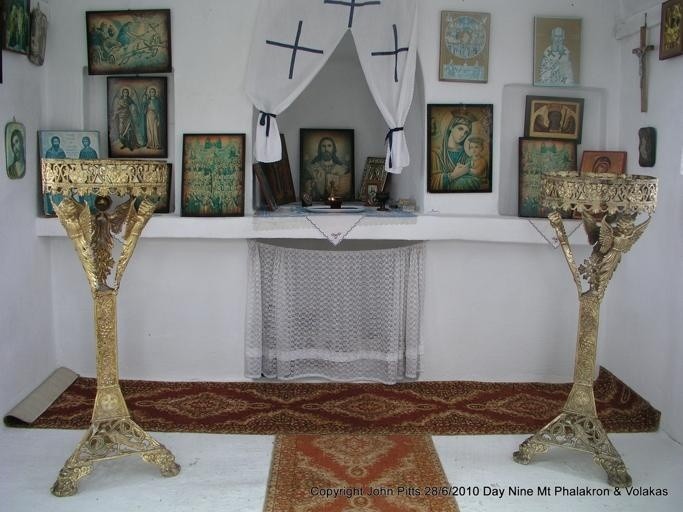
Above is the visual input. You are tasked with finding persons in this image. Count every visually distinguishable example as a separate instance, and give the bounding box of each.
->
[79,137,98,209]
[540,26,575,85]
[431,110,478,190]
[90,21,135,52]
[9,130,26,177]
[113,88,140,151]
[140,88,160,150]
[458,135,488,184]
[592,157,611,174]
[303,136,350,200]
[46,137,66,216]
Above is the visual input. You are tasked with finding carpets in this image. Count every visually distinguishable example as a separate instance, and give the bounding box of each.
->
[3,367,661,434]
[262,434,460,512]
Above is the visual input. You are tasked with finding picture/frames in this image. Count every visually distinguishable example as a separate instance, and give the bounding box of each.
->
[659,0,683,60]
[85,9,245,217]
[298,128,356,202]
[427,10,627,221]
[0,0,31,55]
